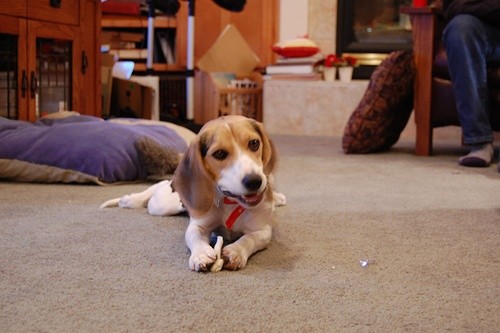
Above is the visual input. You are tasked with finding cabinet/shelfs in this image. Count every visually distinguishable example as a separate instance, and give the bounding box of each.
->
[0,0,188,122]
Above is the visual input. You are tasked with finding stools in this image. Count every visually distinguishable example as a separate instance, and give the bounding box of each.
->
[400,7,500,157]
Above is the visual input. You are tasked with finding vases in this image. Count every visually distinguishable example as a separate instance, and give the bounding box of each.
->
[338,66,353,80]
[322,67,337,80]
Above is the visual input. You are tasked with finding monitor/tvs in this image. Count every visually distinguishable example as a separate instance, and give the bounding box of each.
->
[335,0,413,54]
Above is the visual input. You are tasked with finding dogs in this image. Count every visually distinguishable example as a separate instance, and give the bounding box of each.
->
[96,113,287,275]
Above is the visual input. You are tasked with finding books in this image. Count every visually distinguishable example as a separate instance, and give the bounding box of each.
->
[0,27,185,123]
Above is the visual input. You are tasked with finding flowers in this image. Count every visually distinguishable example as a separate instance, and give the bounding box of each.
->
[314,54,363,68]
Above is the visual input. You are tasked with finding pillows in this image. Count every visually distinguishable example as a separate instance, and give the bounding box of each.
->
[342,51,416,154]
[0,110,198,186]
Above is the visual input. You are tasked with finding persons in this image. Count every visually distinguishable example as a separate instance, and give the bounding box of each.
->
[442,0,500,167]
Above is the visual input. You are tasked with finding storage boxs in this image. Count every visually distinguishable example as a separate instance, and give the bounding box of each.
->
[193,71,264,125]
